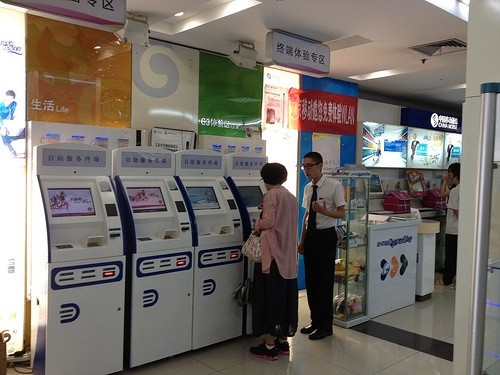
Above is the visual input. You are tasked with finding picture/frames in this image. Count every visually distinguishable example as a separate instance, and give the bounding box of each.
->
[350,170,441,196]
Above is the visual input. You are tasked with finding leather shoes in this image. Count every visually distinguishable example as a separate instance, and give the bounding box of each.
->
[309,326,332,340]
[300,326,316,334]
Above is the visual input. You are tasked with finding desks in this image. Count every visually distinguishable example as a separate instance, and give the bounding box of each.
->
[342,216,419,318]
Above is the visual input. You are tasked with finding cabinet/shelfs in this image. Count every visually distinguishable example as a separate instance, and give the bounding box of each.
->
[327,176,371,329]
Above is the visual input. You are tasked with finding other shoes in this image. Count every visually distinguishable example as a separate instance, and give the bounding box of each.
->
[435,278,444,285]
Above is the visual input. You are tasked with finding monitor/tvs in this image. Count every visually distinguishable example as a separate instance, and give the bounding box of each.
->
[47,188,95,217]
[238,186,263,207]
[186,187,220,209]
[127,187,167,212]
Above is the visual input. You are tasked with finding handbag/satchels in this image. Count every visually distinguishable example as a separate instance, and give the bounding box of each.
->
[241,229,262,263]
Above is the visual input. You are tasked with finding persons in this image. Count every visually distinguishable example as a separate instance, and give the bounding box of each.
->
[0,89,25,157]
[435,162,461,285]
[447,140,454,162]
[410,133,420,160]
[248,163,299,360]
[298,152,346,341]
[377,140,381,163]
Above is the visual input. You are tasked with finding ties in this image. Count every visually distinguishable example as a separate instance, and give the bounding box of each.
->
[307,185,318,237]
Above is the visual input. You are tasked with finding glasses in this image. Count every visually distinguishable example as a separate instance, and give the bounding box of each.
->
[300,163,318,170]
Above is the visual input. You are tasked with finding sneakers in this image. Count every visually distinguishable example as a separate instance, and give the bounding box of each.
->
[275,337,289,354]
[249,340,278,361]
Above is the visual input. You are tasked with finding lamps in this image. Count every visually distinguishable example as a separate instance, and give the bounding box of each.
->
[114,14,149,46]
[229,40,257,70]
